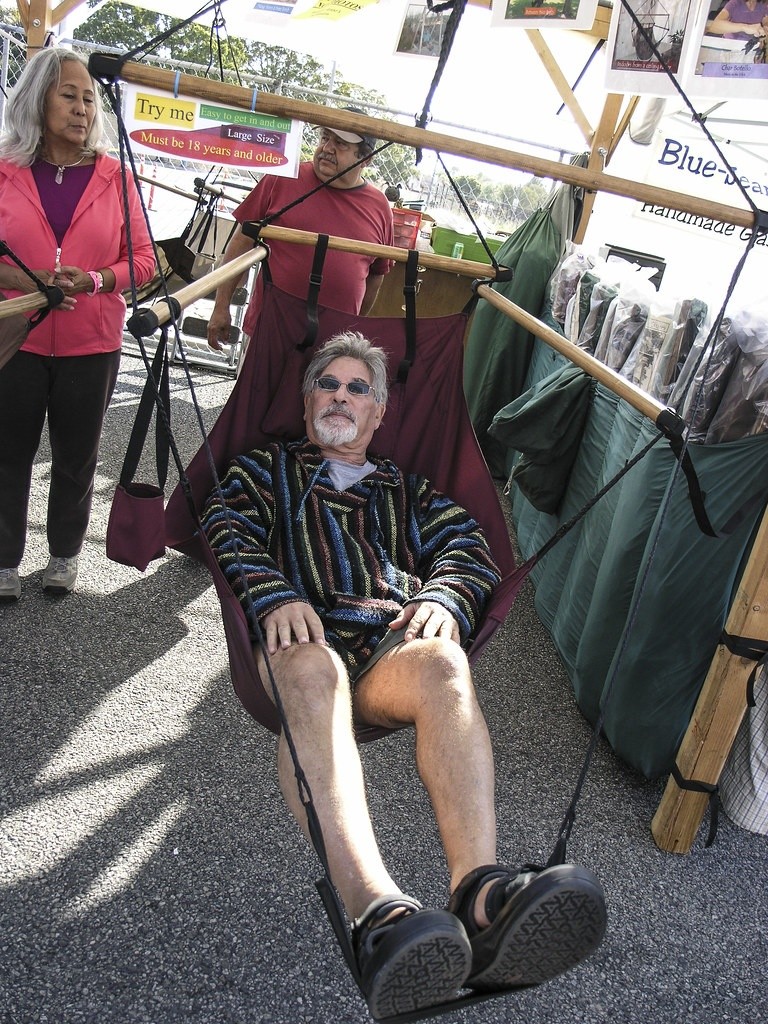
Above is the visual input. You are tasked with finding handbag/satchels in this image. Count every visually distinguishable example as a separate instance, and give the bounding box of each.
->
[105,483,166,572]
[190,253,216,282]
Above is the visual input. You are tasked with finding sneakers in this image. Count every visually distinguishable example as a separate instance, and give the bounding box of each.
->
[42,553,78,595]
[0,568,21,602]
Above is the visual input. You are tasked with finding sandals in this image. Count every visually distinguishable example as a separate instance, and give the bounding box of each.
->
[349,893,472,1014]
[440,863,608,986]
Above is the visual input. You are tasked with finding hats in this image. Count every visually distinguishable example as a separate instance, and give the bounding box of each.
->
[311,107,375,150]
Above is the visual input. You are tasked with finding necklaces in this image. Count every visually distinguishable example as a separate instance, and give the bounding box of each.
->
[37,154,85,184]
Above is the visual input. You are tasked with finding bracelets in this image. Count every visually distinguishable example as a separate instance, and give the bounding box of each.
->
[86,270,104,297]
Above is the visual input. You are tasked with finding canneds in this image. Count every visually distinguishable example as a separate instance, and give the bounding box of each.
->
[451,242,464,259]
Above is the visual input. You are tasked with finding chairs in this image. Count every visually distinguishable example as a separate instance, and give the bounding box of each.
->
[169,211,260,366]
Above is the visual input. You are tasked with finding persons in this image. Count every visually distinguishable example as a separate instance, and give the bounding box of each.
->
[0,48,156,601]
[206,107,395,350]
[710,0,768,41]
[200,331,608,1024]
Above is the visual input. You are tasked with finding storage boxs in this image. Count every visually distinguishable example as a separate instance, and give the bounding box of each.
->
[431,226,504,264]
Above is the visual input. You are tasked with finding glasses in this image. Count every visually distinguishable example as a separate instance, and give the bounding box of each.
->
[313,377,374,395]
